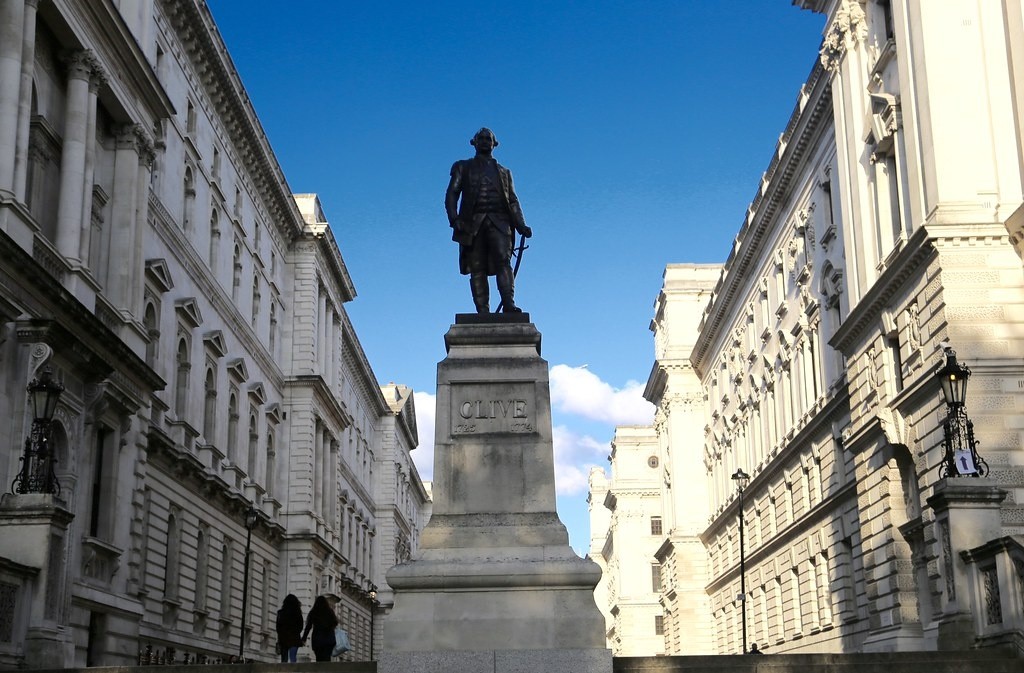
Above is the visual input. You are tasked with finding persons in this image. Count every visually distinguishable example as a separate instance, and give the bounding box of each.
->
[749,643,762,654]
[228,655,255,664]
[276,594,338,662]
[445,128,532,313]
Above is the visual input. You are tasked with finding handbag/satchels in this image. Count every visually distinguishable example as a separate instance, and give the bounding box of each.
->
[276,642,281,655]
[332,626,351,657]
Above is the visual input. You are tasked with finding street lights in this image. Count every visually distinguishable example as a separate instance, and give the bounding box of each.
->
[730,468,751,654]
[368,583,378,661]
[236,499,258,663]
